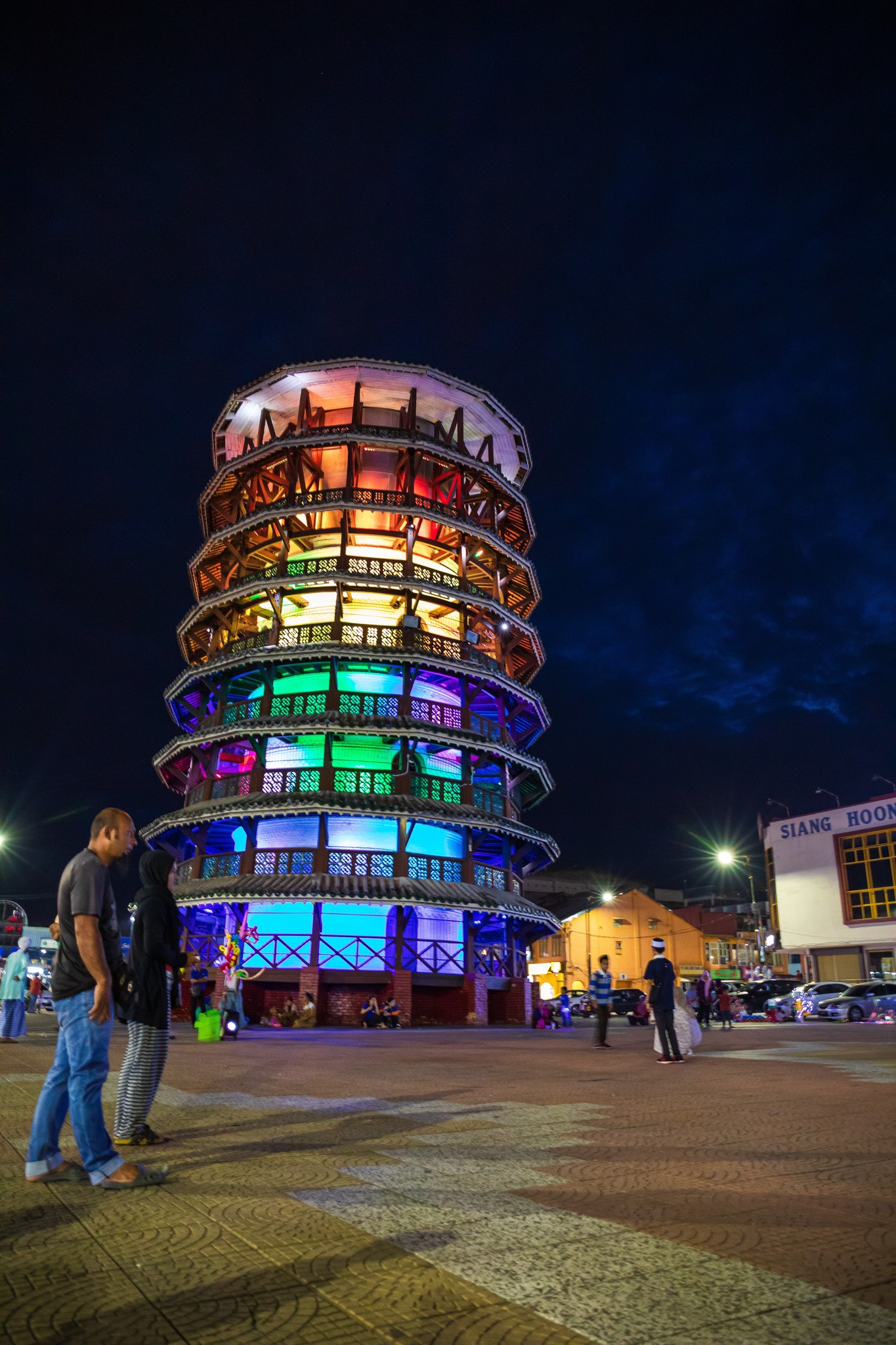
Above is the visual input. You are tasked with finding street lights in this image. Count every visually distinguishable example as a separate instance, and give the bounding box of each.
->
[587,891,612,985]
[717,851,764,980]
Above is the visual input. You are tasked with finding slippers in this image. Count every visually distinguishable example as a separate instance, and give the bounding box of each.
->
[36,1160,89,1183]
[101,1164,166,1190]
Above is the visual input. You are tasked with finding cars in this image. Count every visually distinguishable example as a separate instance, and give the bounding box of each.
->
[817,981,896,1022]
[24,966,54,1012]
[762,981,853,1019]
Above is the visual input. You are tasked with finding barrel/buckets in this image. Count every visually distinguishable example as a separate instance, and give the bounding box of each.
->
[550,1022,556,1030]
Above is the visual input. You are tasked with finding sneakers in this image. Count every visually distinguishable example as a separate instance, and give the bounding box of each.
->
[656,1056,673,1064]
[671,1055,685,1063]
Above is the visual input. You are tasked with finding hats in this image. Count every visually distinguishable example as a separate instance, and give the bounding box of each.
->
[651,937,666,948]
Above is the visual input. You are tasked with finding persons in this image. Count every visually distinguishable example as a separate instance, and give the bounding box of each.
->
[25,807,168,1190]
[635,995,652,1026]
[27,971,43,1013]
[683,970,738,1028]
[794,969,803,981]
[589,955,613,1049]
[190,958,209,1028]
[559,986,574,1029]
[660,981,696,1055]
[218,966,244,1038]
[112,849,203,1145]
[642,938,686,1063]
[256,992,317,1029]
[194,996,212,1022]
[533,999,598,1028]
[360,995,402,1030]
[0,937,31,1044]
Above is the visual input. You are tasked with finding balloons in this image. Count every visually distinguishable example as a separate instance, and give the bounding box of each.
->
[218,910,267,982]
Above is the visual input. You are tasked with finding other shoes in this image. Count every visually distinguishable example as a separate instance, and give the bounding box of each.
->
[113,1123,164,1145]
[592,1043,613,1049]
[0,1037,18,1043]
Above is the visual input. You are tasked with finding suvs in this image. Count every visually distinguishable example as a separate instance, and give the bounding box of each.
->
[729,978,806,1015]
[543,989,590,1016]
[683,980,751,997]
[579,988,648,1018]
